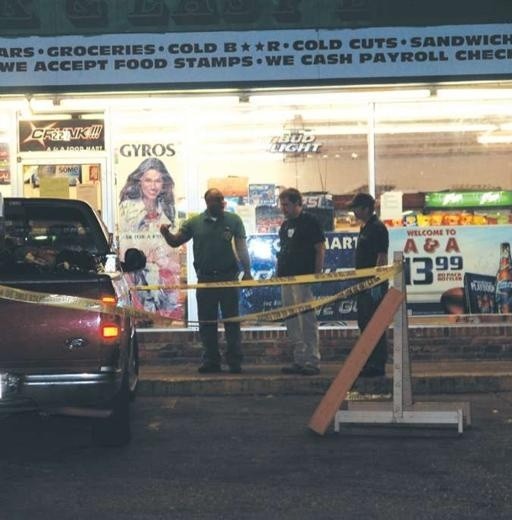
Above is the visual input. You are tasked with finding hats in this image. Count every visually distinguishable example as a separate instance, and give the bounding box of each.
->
[349,192,376,208]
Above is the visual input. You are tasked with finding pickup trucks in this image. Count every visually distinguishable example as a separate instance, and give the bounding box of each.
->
[1,197,148,449]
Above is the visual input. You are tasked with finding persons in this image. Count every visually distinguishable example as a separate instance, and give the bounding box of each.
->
[116,156,179,323]
[273,187,326,377]
[160,187,253,372]
[348,192,390,378]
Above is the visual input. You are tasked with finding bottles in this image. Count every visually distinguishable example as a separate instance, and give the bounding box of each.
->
[493,242,511,314]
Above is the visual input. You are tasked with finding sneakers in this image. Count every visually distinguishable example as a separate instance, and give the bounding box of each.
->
[280,361,321,375]
[228,359,242,372]
[358,364,387,378]
[197,359,222,375]
